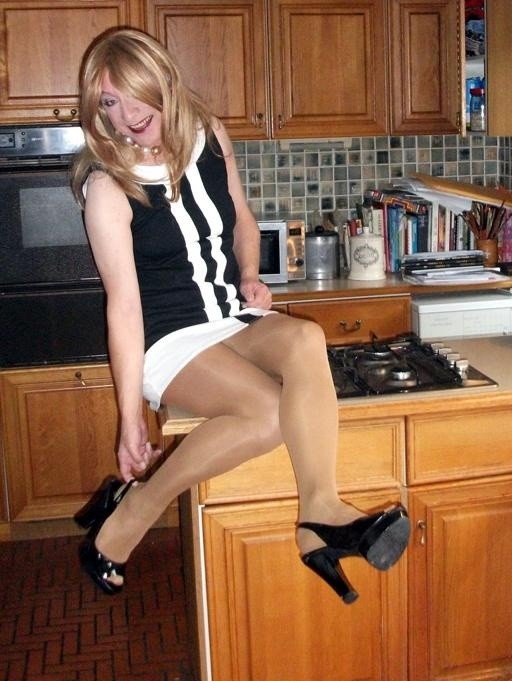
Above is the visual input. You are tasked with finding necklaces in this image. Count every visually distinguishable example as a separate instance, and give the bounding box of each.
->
[117,136,163,158]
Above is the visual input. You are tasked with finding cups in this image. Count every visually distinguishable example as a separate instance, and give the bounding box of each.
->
[476,238,498,267]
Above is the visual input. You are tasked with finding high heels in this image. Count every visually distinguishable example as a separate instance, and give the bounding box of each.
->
[74,474,136,594]
[288,508,410,605]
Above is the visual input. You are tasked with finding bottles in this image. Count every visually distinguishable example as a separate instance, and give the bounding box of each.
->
[469,89,487,133]
[346,227,388,281]
[306,227,341,279]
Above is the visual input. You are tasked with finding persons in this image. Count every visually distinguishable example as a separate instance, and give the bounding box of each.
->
[74,29,411,605]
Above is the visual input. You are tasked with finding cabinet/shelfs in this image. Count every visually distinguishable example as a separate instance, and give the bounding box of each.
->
[388,1,512,137]
[1,366,183,540]
[136,1,388,142]
[269,288,417,350]
[0,0,136,130]
[174,392,512,680]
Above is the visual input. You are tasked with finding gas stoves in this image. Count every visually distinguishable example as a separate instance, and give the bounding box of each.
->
[330,332,499,402]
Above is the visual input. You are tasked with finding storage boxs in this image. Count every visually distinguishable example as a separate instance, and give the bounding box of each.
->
[410,289,511,341]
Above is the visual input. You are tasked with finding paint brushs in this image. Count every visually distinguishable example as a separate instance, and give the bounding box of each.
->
[458,199,511,239]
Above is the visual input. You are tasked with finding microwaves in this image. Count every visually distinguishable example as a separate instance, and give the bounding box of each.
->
[255,220,307,285]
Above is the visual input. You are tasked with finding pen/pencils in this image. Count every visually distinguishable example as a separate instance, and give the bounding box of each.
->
[348,203,363,237]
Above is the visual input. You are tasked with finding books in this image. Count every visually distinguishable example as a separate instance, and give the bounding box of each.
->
[356,171,512,285]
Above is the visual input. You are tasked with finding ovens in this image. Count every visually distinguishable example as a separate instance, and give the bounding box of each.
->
[0,125,109,373]
